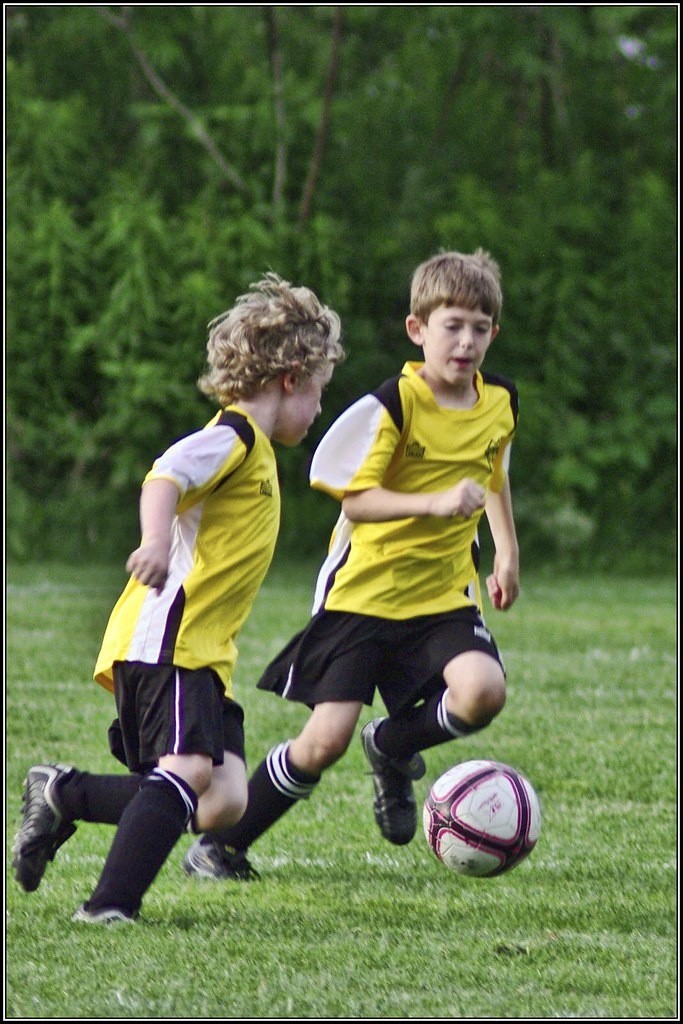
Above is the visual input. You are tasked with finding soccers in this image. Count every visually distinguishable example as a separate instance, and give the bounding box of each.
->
[422,758,541,879]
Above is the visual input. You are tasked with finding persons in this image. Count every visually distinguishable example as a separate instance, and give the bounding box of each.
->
[13,280,350,925]
[183,253,520,881]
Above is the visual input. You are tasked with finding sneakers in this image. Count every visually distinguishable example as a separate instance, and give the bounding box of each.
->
[184,831,257,883]
[71,902,135,929]
[13,761,80,893]
[361,716,427,845]
